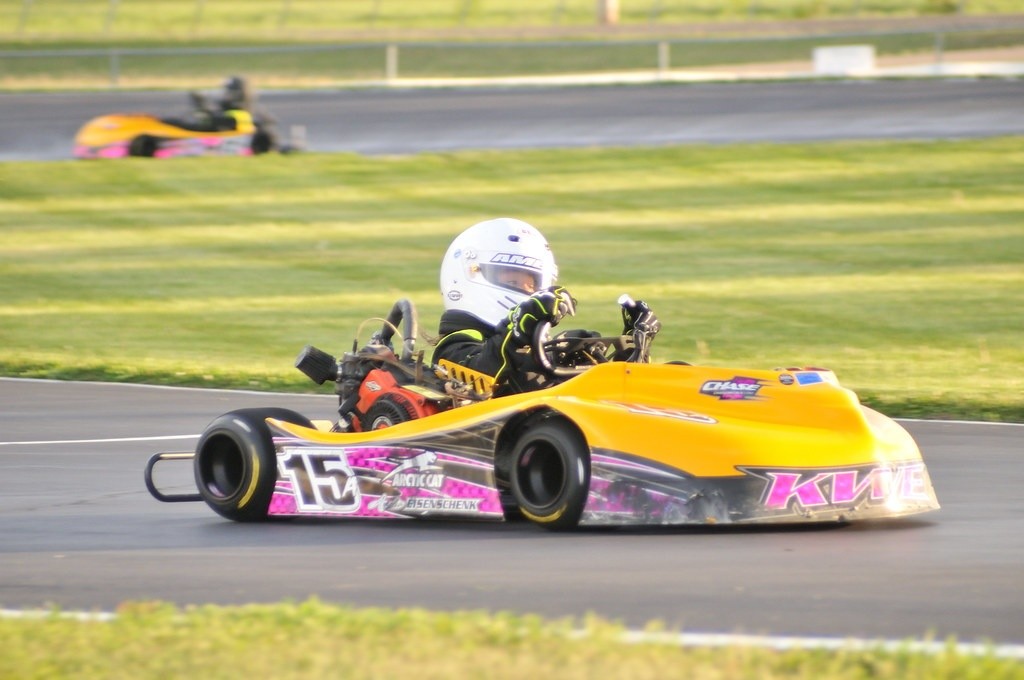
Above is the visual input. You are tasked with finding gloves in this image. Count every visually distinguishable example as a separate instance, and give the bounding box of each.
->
[622,300,661,340]
[508,286,577,340]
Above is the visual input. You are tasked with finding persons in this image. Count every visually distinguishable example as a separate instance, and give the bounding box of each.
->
[431,218,661,402]
[194,75,270,139]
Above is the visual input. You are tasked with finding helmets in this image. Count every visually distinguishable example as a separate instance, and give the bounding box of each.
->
[440,217,558,328]
